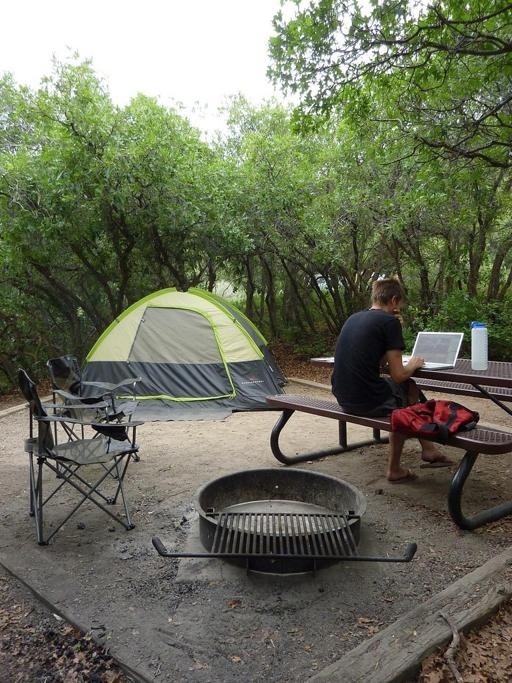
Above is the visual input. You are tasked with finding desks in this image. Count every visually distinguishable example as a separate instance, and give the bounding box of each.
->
[311,346,512,449]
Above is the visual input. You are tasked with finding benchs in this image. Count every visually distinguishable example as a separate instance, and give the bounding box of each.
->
[265,373,510,531]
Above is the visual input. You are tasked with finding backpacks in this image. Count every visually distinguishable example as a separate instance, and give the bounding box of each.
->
[390,398,480,441]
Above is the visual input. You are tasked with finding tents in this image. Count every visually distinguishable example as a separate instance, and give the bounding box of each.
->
[81,287,288,422]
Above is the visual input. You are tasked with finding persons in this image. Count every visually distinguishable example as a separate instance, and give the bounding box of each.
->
[331,277,457,484]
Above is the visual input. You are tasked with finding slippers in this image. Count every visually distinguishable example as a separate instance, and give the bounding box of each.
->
[419,452,459,469]
[386,467,417,485]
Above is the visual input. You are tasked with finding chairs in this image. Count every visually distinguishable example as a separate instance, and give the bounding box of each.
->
[16,353,144,546]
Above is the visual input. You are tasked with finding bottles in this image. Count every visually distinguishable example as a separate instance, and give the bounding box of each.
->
[469,319,489,371]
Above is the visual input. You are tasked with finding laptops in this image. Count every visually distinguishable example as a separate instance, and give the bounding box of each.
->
[386,332,465,371]
